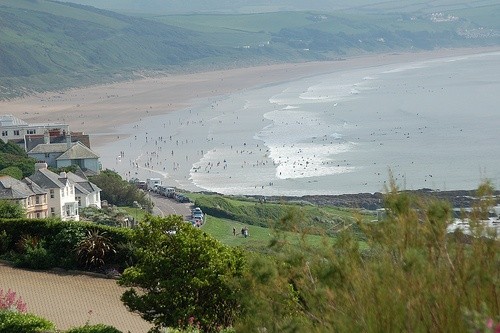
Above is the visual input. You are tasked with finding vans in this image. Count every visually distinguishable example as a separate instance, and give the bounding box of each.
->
[138,181,146,189]
[153,184,191,203]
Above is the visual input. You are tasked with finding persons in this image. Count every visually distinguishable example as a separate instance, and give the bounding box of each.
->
[229,224,250,239]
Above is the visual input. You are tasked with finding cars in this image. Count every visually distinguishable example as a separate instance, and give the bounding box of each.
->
[189,207,204,228]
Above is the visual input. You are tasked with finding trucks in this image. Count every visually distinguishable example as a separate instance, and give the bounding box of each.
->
[145,179,161,192]
